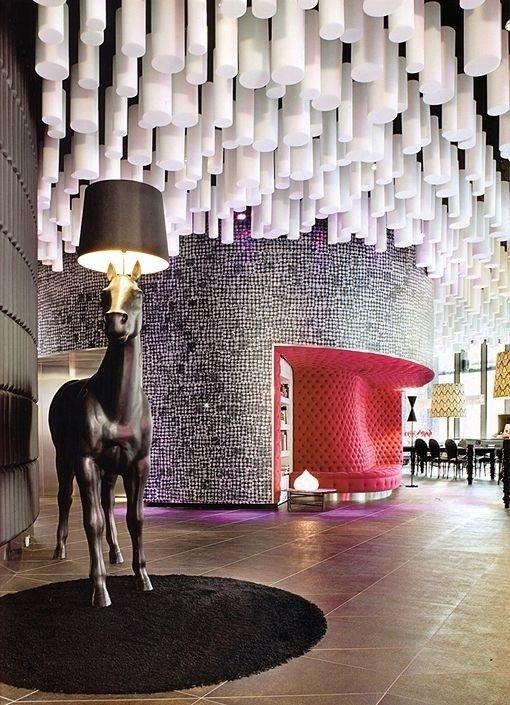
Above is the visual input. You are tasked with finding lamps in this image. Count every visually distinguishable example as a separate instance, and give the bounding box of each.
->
[76,180,171,276]
[406,397,418,487]
[293,470,320,492]
[493,351,510,398]
[430,383,467,441]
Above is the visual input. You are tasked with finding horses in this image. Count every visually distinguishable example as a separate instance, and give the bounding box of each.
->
[46,257,156,611]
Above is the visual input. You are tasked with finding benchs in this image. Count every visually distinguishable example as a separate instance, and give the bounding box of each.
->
[290,464,403,502]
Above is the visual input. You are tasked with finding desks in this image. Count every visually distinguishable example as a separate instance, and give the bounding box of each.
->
[285,488,337,512]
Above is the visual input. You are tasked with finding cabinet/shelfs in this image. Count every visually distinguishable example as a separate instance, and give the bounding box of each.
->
[277,358,293,505]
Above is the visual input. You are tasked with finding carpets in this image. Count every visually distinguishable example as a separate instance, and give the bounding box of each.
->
[0,573,328,694]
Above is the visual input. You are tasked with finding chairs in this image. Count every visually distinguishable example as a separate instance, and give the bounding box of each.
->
[415,439,502,480]
